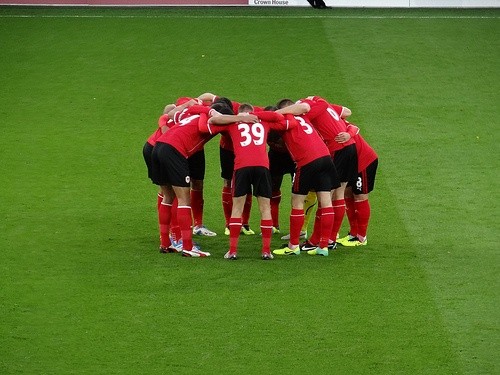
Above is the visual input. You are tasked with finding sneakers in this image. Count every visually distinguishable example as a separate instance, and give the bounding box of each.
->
[241,225,255,235]
[281,231,307,239]
[159,238,182,253]
[259,227,280,236]
[182,246,210,257]
[307,247,328,257]
[273,246,300,255]
[337,232,368,246]
[301,239,318,250]
[328,242,337,250]
[193,225,217,236]
[223,251,236,259]
[225,227,230,236]
[262,252,274,259]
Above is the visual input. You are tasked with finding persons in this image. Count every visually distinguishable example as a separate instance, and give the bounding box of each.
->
[143,93,358,260]
[334,119,379,247]
